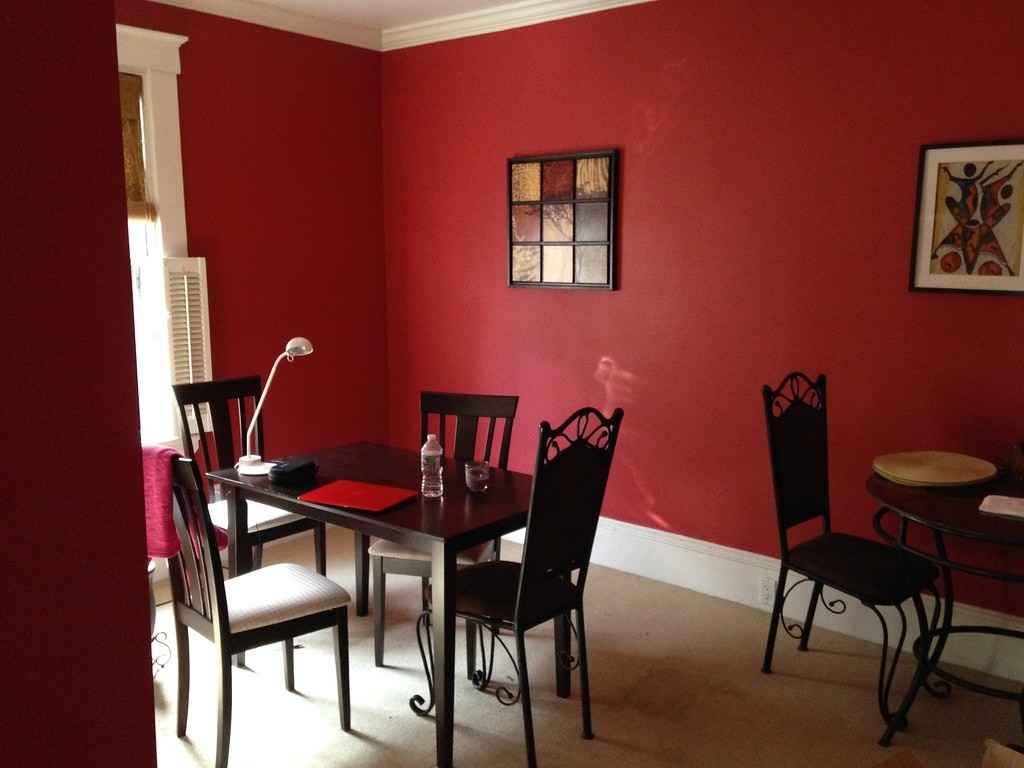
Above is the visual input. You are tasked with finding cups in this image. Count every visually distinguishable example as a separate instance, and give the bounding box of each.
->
[465,460,488,492]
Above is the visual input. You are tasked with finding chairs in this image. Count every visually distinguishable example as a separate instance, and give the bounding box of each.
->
[167,456,351,768]
[761,372,940,732]
[410,407,623,768]
[367,390,518,680]
[172,375,326,577]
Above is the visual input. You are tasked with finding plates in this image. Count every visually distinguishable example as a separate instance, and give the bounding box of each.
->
[871,451,997,487]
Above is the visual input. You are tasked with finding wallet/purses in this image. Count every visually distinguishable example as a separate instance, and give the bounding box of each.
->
[268,461,317,483]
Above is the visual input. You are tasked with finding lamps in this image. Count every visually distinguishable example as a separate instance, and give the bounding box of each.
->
[236,336,314,475]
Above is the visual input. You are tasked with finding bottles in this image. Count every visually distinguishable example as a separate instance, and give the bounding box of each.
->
[422,435,444,498]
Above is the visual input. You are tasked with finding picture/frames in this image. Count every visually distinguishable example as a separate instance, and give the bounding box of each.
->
[505,148,618,290]
[909,139,1024,297]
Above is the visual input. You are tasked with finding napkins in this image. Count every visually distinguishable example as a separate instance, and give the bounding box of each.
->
[979,494,1024,518]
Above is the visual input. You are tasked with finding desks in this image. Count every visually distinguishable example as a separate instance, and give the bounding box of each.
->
[865,467,1024,749]
[206,441,534,768]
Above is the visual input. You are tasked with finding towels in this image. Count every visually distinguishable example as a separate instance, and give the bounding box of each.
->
[141,444,229,595]
